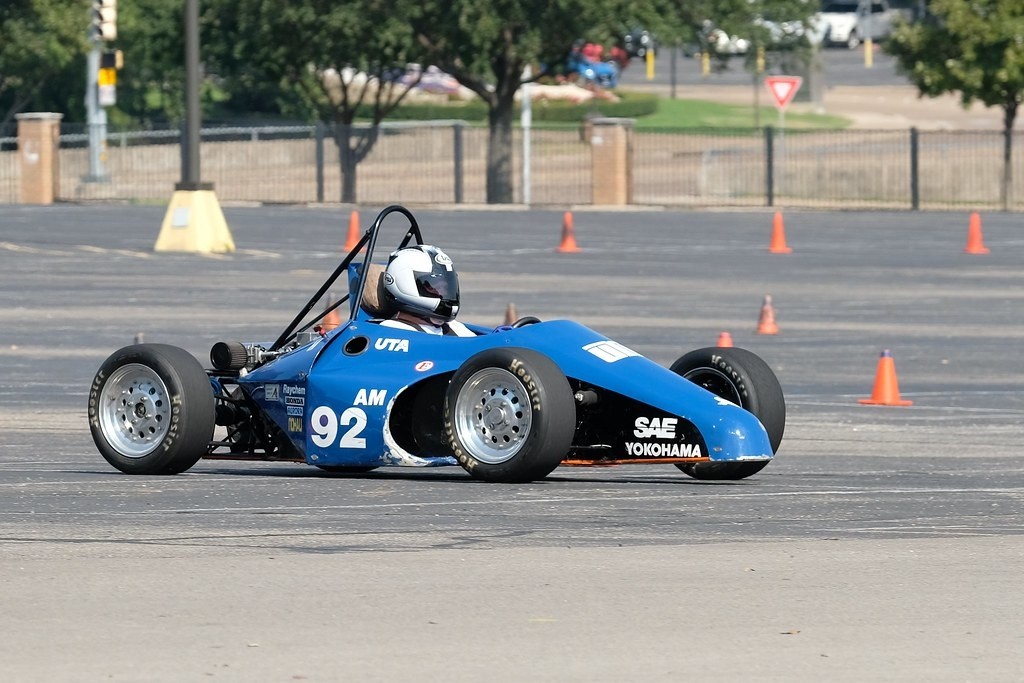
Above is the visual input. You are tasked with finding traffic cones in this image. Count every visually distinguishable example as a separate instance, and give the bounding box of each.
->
[501,303,517,325]
[343,212,368,252]
[767,213,792,253]
[966,213,989,253]
[318,293,341,331]
[717,331,736,348]
[857,348,915,407]
[558,209,585,252]
[757,295,780,334]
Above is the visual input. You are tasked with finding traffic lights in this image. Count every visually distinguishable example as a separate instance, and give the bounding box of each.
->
[99,50,122,68]
[93,0,119,44]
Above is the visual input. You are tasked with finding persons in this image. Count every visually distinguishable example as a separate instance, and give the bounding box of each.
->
[379,244,478,338]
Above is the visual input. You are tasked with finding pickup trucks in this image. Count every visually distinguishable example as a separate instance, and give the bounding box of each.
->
[808,0,915,51]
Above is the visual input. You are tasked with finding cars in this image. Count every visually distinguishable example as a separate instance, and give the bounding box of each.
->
[622,30,656,61]
[699,19,805,59]
[572,41,629,78]
[541,53,618,89]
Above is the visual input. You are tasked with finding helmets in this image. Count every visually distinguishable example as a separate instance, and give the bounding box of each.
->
[383,244,459,322]
